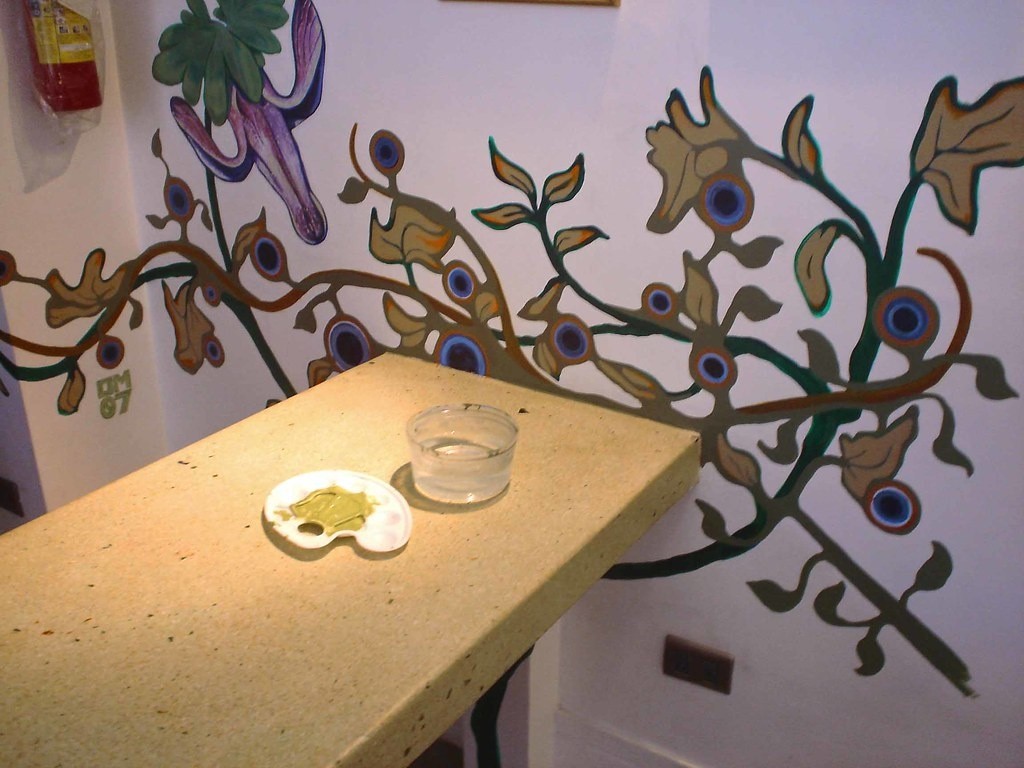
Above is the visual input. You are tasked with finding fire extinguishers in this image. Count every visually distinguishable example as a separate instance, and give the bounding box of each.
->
[23,0,103,111]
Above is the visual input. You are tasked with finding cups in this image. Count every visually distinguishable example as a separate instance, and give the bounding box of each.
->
[407,403,519,503]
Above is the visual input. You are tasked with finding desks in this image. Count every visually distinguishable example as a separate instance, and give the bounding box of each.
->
[0,351,702,768]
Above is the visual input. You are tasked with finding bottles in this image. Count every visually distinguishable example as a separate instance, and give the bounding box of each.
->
[22,0,103,111]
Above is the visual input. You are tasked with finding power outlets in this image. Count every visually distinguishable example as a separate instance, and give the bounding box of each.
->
[662,634,735,695]
